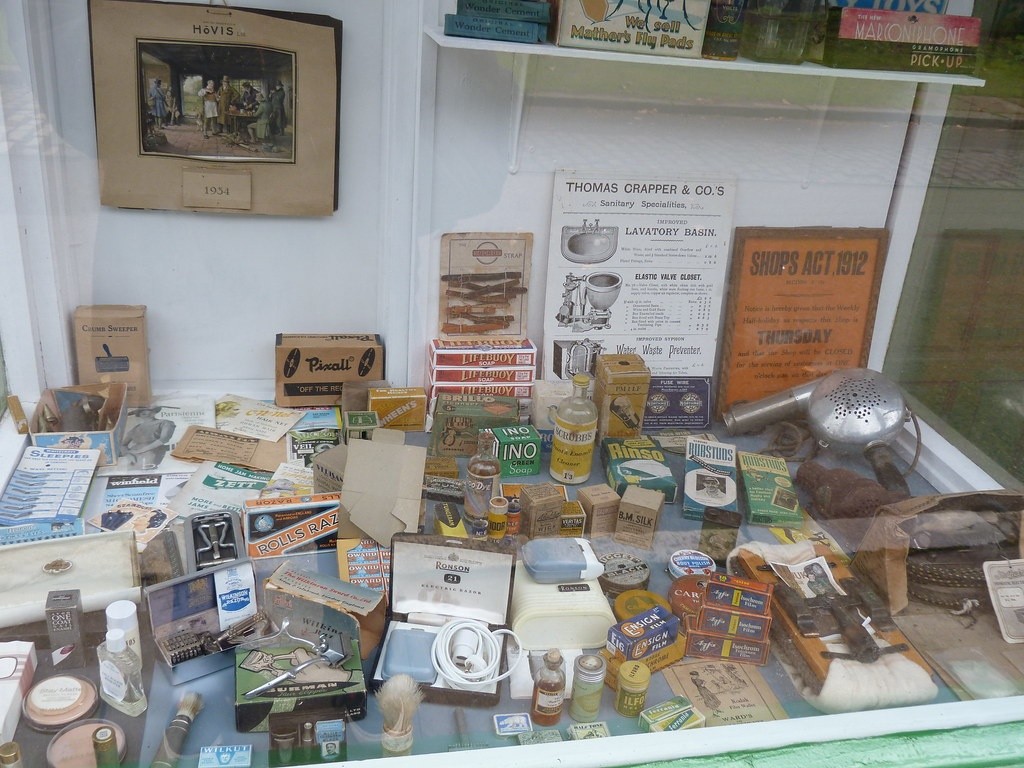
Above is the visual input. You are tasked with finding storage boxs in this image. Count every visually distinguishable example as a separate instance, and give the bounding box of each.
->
[684,614,768,668]
[613,486,665,548]
[531,378,594,451]
[72,304,151,408]
[561,500,584,538]
[275,334,384,407]
[431,394,517,459]
[369,389,425,431]
[522,485,566,541]
[236,637,367,732]
[369,532,516,706]
[550,2,709,59]
[702,572,773,617]
[605,605,680,664]
[425,475,465,502]
[500,483,568,503]
[580,484,620,538]
[145,556,279,685]
[593,355,650,440]
[28,382,127,468]
[425,457,459,478]
[457,0,549,25]
[444,13,545,44]
[737,450,805,530]
[607,632,686,685]
[345,411,377,445]
[484,426,540,476]
[697,605,772,640]
[435,502,469,538]
[426,338,537,420]
[313,443,348,491]
[45,589,86,669]
[807,8,985,77]
[681,436,738,525]
[241,490,342,557]
[649,707,705,729]
[600,432,675,504]
[0,642,38,746]
[639,695,693,732]
[265,561,388,661]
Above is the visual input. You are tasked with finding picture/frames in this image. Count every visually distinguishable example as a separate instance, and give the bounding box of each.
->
[910,230,1023,382]
[715,227,888,432]
[1,529,184,647]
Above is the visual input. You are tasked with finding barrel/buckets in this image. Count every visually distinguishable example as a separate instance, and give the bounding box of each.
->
[738,0,829,65]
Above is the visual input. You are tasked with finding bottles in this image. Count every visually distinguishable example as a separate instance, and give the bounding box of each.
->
[530,649,567,726]
[43,404,61,433]
[91,725,120,767]
[700,0,747,60]
[570,655,606,723]
[488,495,508,538]
[614,661,651,717]
[0,742,25,768]
[549,374,598,485]
[506,501,520,538]
[106,600,143,669]
[97,629,147,718]
[464,434,500,526]
[471,518,488,542]
